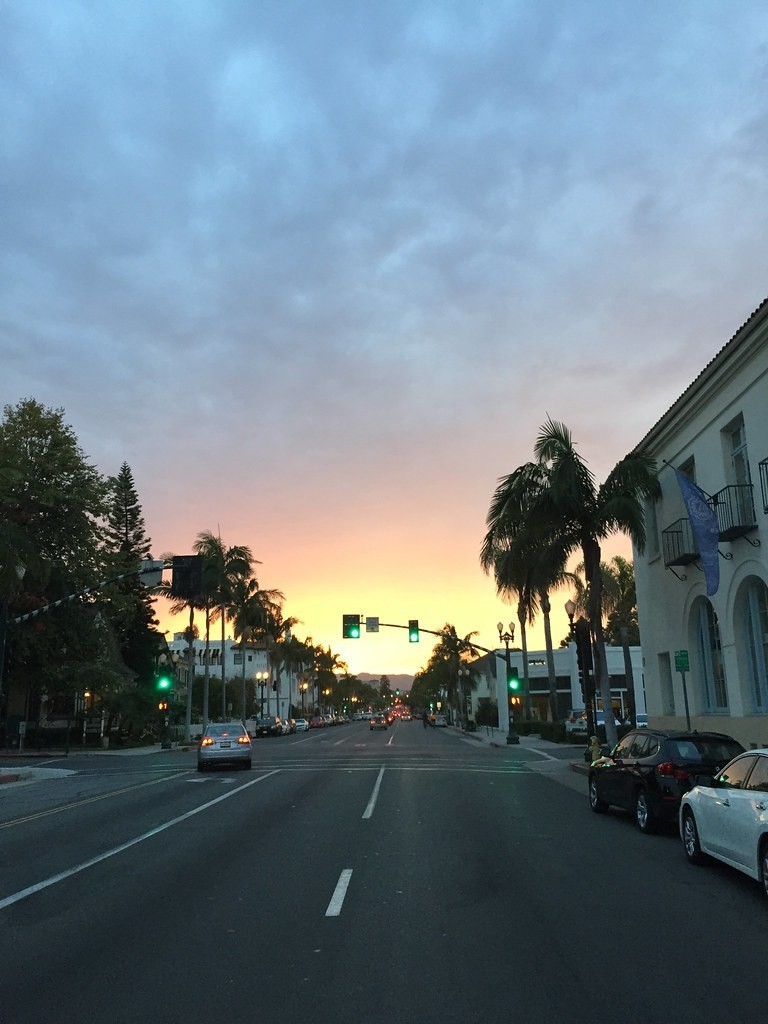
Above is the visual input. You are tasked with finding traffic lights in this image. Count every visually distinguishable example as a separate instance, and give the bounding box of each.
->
[342,615,360,639]
[396,689,400,696]
[508,666,520,696]
[577,672,586,702]
[158,702,168,713]
[159,668,171,695]
[510,698,519,707]
[408,619,420,643]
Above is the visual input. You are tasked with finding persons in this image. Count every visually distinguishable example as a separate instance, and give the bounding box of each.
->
[422,712,429,729]
[431,712,436,727]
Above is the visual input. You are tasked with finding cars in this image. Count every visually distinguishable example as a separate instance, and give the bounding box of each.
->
[370,716,388,730]
[198,723,253,770]
[295,718,310,731]
[354,707,413,725]
[429,715,448,728]
[254,716,296,737]
[588,729,749,833]
[679,747,768,889]
[310,714,349,728]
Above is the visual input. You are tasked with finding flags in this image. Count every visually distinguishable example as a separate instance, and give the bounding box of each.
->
[675,469,720,597]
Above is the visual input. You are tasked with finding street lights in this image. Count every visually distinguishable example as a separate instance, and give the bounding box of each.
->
[496,621,521,744]
[256,671,270,718]
[458,669,471,730]
[159,652,180,748]
[299,683,309,718]
